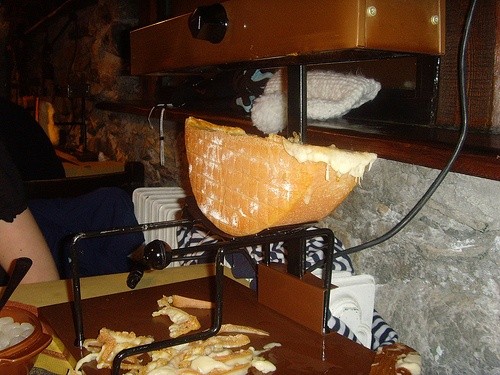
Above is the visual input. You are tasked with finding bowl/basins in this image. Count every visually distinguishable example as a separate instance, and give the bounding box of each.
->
[0,306,53,375]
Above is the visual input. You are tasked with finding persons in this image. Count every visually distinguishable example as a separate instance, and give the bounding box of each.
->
[0,156,61,285]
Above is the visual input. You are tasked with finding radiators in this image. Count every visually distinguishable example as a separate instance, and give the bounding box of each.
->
[133,186,374,350]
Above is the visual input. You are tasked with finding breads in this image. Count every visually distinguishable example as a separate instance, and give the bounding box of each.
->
[74,294,423,375]
[186,115,377,237]
